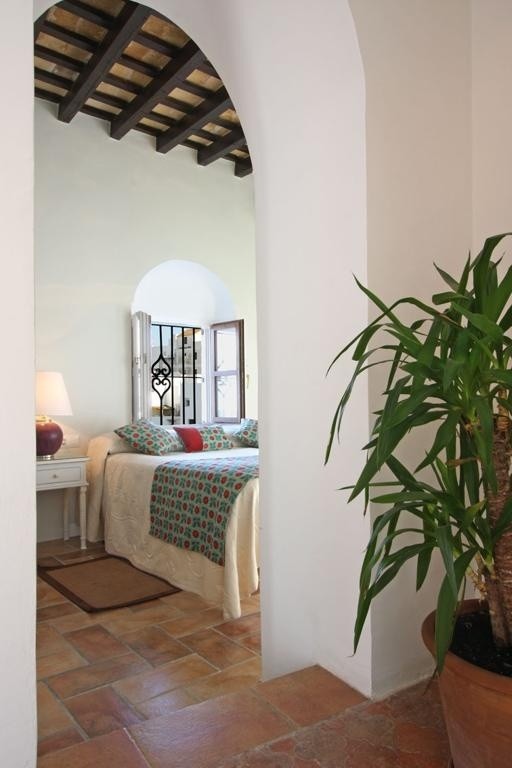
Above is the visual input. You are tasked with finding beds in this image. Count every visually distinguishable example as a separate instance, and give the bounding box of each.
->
[100,445,259,622]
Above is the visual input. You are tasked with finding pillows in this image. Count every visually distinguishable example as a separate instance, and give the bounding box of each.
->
[93,417,258,454]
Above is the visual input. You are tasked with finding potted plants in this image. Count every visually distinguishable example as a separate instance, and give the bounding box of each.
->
[322,235,511,768]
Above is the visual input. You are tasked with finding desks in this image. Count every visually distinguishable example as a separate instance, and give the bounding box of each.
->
[36,453,91,552]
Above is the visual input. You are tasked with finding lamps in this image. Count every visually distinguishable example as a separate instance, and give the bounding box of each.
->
[36,371,72,459]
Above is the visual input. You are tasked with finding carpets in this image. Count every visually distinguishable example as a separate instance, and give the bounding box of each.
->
[39,551,181,613]
